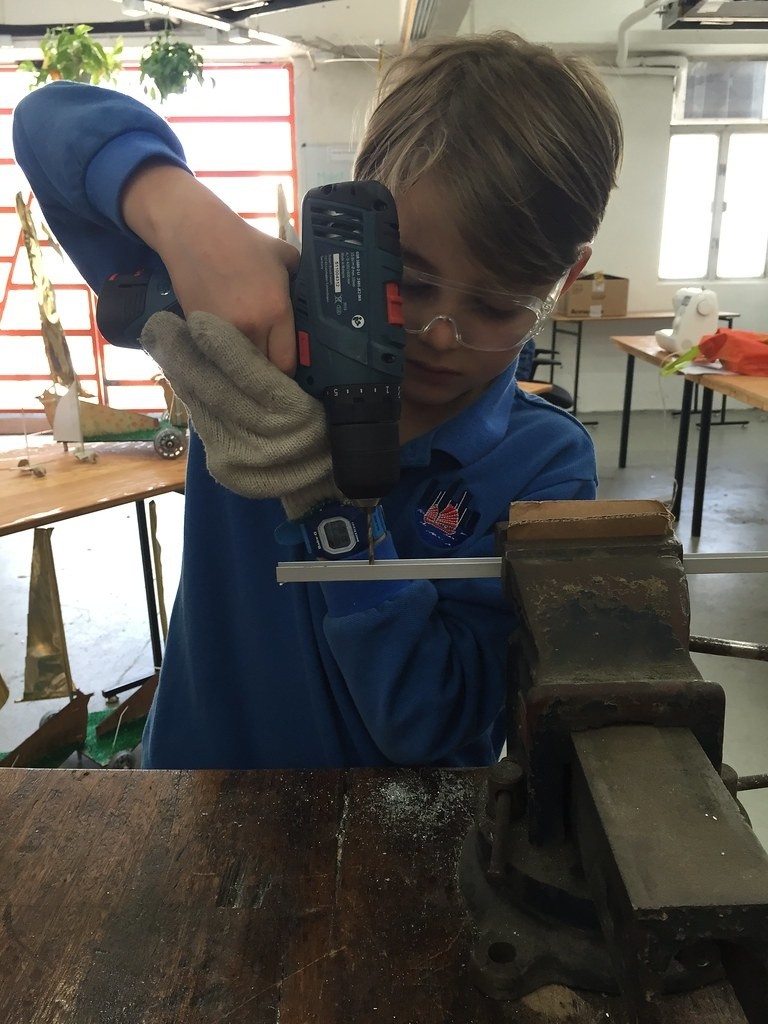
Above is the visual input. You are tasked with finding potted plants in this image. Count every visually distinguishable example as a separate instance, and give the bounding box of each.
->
[138,34,208,100]
[16,23,125,82]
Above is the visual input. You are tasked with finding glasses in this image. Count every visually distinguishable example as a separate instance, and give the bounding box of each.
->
[398,242,595,351]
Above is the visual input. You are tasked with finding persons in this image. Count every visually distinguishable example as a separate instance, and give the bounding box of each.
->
[12,32,624,769]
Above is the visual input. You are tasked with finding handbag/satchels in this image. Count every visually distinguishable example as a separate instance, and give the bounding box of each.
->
[660,327,768,380]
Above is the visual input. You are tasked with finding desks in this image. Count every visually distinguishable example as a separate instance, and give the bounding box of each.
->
[1,765,751,1023]
[609,334,768,538]
[0,437,188,702]
[548,310,739,428]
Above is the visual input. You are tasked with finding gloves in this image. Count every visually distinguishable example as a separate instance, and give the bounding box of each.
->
[139,308,347,520]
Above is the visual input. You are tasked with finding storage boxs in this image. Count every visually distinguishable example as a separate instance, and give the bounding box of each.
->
[557,272,629,317]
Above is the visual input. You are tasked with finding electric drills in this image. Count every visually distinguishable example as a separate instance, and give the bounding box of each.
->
[99,180,414,565]
